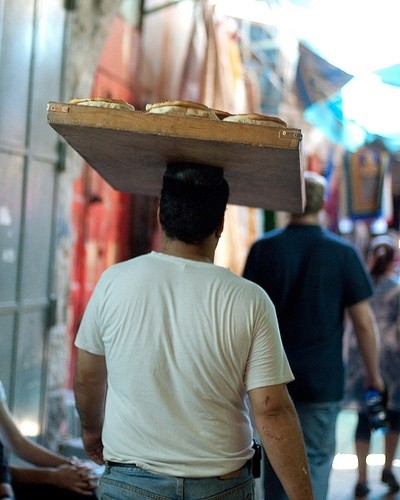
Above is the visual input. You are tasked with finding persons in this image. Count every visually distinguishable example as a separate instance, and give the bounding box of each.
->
[348,191,400,500]
[73,153,315,500]
[241,170,386,500]
[0,379,98,500]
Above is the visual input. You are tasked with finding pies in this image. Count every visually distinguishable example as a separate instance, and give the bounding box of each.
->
[68,97,288,128]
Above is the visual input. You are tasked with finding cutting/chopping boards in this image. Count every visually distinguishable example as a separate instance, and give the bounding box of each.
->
[46,101,308,215]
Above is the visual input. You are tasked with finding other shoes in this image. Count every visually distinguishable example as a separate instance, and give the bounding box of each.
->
[382,469,400,491]
[355,484,369,497]
